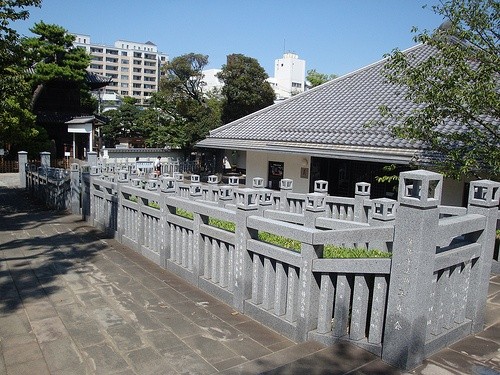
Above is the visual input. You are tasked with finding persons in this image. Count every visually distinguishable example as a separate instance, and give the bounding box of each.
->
[154,155,162,177]
[222,157,232,173]
